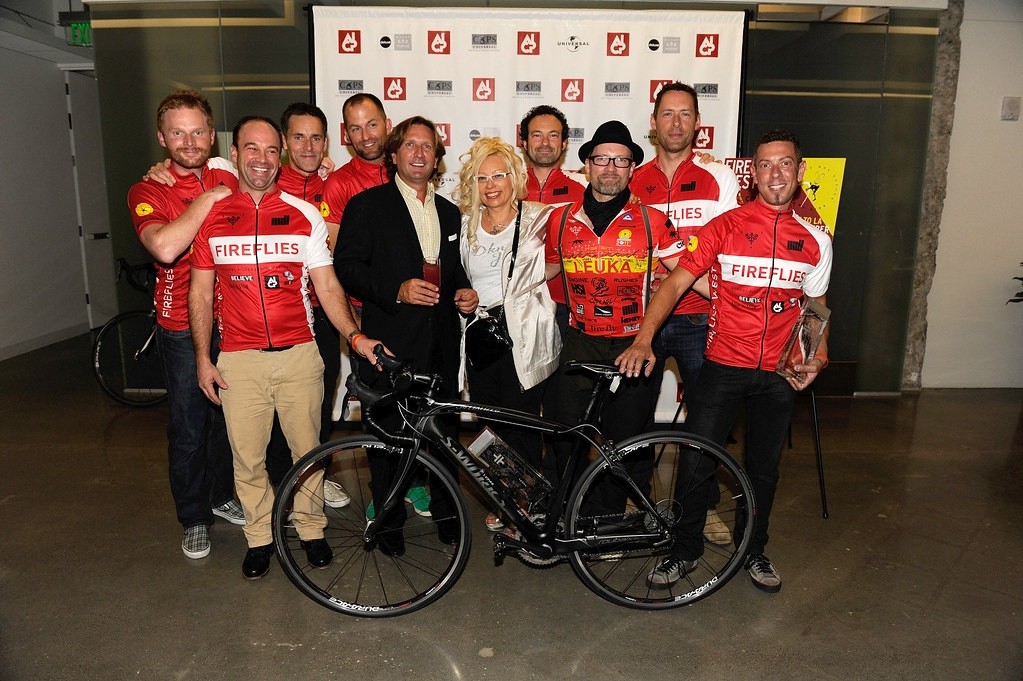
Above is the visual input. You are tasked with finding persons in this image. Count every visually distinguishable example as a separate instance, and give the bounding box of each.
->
[189,116,395,580]
[520,106,722,475]
[333,116,479,557]
[142,102,351,508]
[615,128,834,590]
[629,83,740,546]
[127,90,336,559]
[320,93,393,331]
[544,120,710,526]
[454,136,642,529]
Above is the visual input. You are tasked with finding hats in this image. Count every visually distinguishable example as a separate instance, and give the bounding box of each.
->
[577,120,645,167]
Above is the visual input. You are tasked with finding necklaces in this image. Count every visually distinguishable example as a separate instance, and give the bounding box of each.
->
[487,215,506,235]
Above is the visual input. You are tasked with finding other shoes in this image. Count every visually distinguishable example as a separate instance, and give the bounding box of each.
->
[181,524,213,559]
[599,550,624,562]
[484,511,505,531]
[212,500,248,525]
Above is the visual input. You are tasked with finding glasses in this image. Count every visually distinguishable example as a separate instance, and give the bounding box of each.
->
[588,155,632,168]
[472,172,512,184]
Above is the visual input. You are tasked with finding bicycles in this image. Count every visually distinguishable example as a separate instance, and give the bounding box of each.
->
[270,341,759,618]
[91,257,167,409]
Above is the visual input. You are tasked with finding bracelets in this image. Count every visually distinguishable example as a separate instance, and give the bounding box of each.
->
[815,353,828,368]
[348,330,366,350]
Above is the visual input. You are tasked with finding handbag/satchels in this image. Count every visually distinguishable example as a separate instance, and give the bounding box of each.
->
[463,316,514,373]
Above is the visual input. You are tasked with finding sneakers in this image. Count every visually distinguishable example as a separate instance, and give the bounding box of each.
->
[364,491,376,520]
[274,504,327,528]
[300,538,335,569]
[742,552,782,592]
[241,541,276,580]
[701,510,733,545]
[645,556,698,590]
[404,485,434,516]
[321,479,351,507]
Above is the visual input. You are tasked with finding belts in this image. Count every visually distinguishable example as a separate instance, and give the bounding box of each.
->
[255,345,296,352]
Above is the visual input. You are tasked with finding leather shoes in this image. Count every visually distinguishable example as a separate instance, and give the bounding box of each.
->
[428,499,461,545]
[375,515,406,557]
